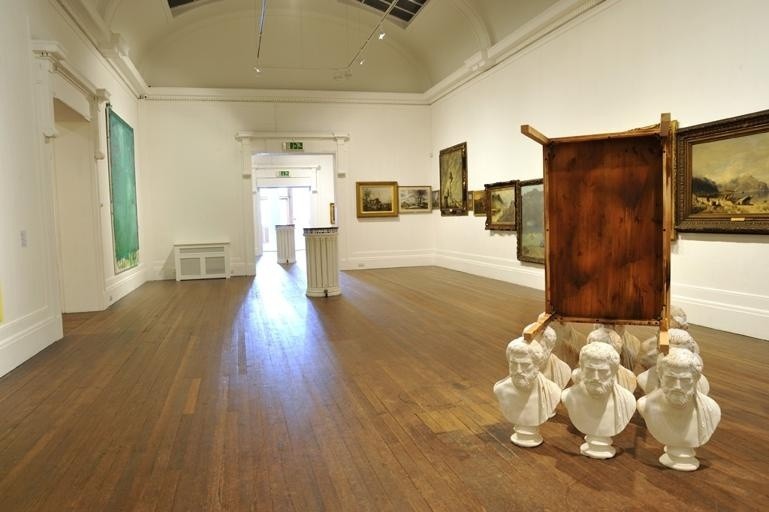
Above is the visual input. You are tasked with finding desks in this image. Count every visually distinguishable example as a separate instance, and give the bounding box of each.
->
[519,112,680,355]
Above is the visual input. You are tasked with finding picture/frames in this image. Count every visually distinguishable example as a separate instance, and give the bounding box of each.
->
[485,180,519,231]
[432,191,440,208]
[330,203,335,224]
[438,141,467,214]
[356,181,398,218]
[677,113,769,237]
[474,191,487,216]
[467,191,473,209]
[397,187,432,214]
[519,181,546,265]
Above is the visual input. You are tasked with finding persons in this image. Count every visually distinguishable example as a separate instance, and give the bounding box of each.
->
[489,304,723,474]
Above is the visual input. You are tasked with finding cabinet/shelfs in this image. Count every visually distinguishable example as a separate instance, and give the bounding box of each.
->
[172,240,233,282]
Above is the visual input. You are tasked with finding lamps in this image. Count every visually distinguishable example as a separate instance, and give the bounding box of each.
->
[346,0,399,69]
